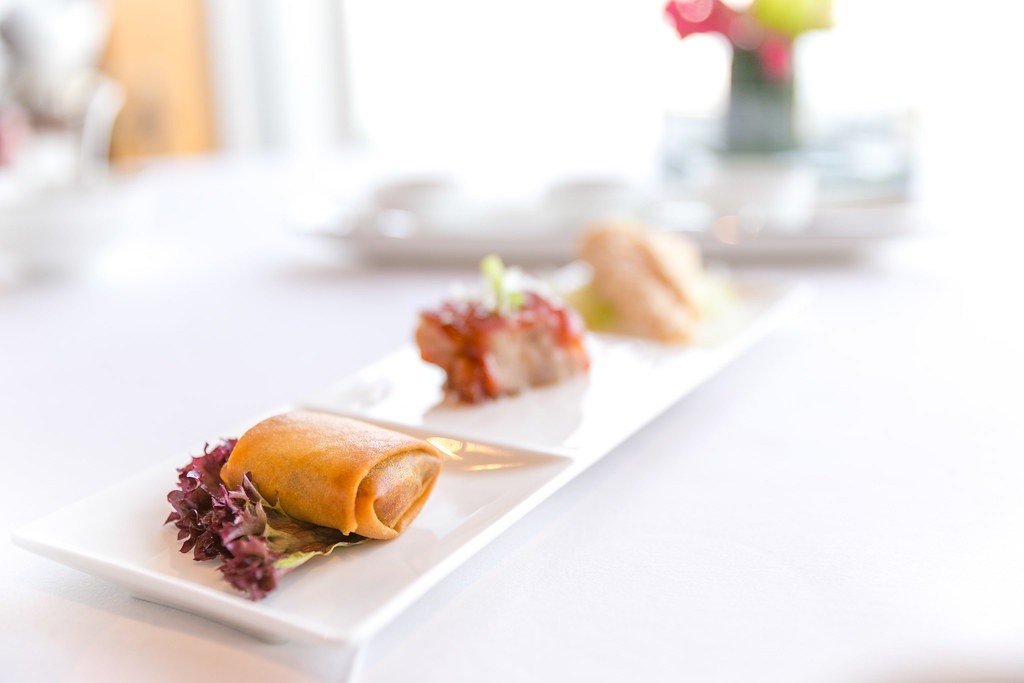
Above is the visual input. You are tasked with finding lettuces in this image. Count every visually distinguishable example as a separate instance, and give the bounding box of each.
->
[164,436,374,600]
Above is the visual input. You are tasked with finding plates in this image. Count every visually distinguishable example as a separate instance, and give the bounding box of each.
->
[14,284,818,647]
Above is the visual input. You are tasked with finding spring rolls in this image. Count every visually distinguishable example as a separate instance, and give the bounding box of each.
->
[220,412,446,543]
